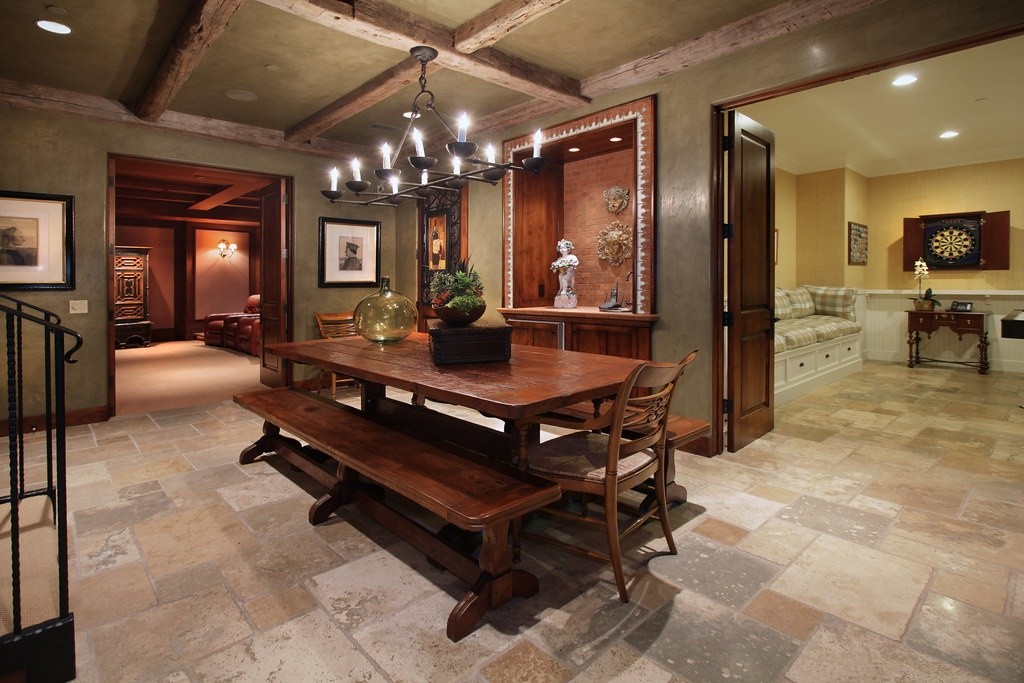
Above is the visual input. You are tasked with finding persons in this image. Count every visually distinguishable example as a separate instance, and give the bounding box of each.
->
[550,239,579,295]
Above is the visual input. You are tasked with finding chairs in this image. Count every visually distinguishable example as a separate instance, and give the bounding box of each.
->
[512,348,700,602]
[313,311,358,401]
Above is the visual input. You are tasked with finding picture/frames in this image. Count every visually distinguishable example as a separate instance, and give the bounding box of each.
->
[775,229,779,265]
[0,190,76,291]
[318,217,381,288]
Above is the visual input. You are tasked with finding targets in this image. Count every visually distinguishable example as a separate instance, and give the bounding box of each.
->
[927,226,976,262]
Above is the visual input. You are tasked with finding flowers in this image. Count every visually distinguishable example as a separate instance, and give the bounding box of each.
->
[907,257,942,307]
[423,254,486,316]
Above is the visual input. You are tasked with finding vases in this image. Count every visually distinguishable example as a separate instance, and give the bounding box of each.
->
[913,301,935,311]
[432,305,486,326]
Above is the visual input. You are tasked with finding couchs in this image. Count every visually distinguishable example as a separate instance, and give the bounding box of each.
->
[204,313,261,357]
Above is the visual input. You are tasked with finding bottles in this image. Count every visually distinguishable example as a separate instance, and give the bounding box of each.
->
[352,276,419,345]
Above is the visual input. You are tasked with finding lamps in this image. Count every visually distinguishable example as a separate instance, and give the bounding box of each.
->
[320,46,546,208]
[218,237,237,257]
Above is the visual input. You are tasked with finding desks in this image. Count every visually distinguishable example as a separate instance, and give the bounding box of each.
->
[264,331,650,567]
[905,310,993,374]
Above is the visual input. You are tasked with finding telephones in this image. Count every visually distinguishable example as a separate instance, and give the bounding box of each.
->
[951,301,973,312]
[599,282,625,311]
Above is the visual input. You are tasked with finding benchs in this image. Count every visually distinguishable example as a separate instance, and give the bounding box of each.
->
[411,392,711,524]
[724,285,863,408]
[234,386,562,642]
